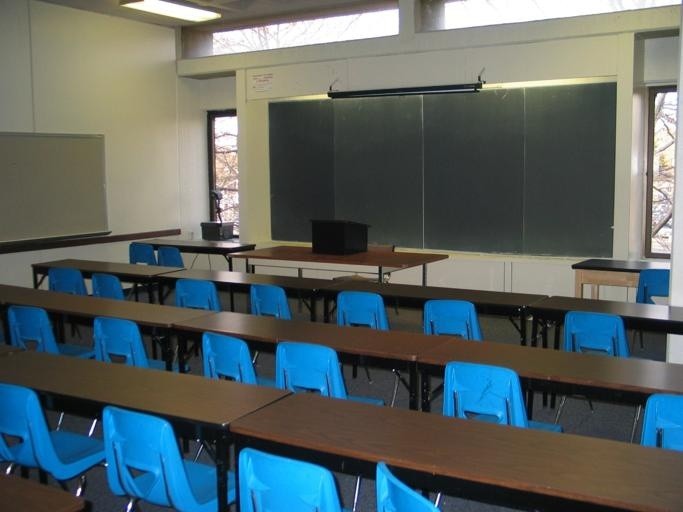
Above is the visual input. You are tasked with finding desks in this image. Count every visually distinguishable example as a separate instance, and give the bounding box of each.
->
[133,239,257,312]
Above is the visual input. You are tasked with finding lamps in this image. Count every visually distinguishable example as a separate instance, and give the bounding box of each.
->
[120,1,225,24]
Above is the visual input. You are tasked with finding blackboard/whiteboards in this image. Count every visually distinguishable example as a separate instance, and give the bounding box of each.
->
[0,132,113,244]
